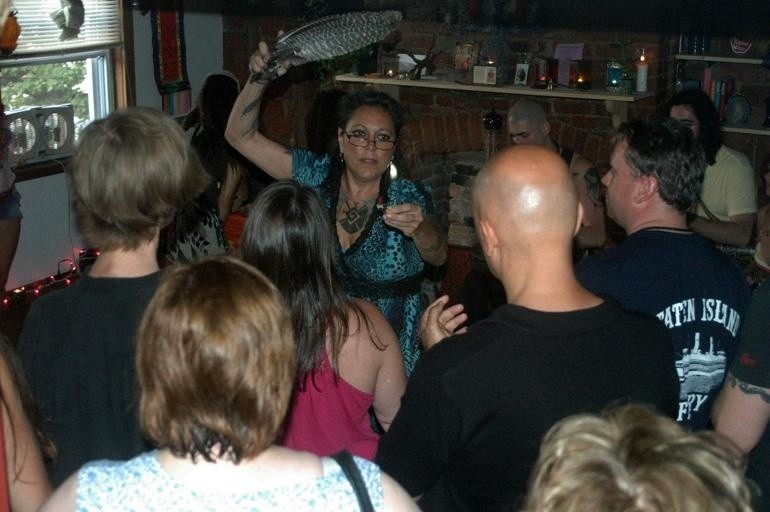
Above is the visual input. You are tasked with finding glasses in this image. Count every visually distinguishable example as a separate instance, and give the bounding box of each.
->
[343,129,396,151]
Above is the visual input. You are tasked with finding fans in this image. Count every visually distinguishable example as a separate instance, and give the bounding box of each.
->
[4,103,77,167]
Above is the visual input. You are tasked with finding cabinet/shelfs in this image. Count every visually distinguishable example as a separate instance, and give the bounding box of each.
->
[673,53,770,138]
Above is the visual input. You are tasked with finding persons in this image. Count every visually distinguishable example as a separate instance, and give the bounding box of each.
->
[0,42,770,512]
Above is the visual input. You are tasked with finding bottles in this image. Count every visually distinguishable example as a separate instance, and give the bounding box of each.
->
[635,47,648,93]
